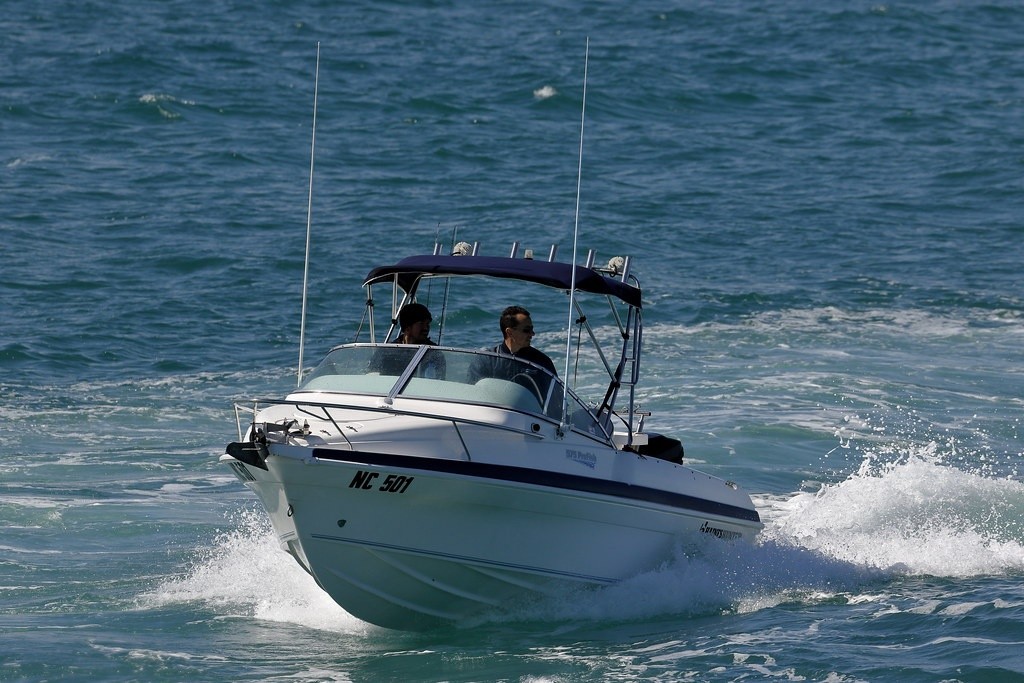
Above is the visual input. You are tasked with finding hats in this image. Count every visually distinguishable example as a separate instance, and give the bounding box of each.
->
[399,304,431,332]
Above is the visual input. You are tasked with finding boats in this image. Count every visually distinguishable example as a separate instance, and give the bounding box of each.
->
[216,33,766,633]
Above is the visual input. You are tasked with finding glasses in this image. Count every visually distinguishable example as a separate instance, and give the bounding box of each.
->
[513,325,534,332]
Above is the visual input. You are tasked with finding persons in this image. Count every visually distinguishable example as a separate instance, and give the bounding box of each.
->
[363,303,446,381]
[466,306,563,421]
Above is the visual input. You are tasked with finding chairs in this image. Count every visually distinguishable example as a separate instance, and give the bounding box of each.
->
[568,407,613,441]
